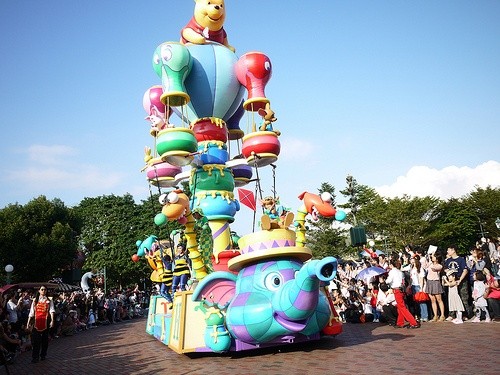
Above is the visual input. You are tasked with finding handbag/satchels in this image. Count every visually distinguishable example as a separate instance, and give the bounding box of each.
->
[414,292,430,303]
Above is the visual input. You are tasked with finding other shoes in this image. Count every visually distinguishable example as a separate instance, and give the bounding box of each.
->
[168,302,173,309]
[32,357,38,363]
[446,316,453,320]
[6,352,16,358]
[420,317,428,322]
[452,319,463,324]
[463,315,468,320]
[6,356,15,364]
[41,356,45,360]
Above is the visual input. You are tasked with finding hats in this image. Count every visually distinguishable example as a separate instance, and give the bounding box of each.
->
[410,254,420,260]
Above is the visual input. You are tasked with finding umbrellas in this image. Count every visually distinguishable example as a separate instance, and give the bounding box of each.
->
[412,291,430,303]
[354,265,387,294]
[360,248,384,260]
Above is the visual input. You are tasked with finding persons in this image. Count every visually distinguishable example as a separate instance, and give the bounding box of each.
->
[324,233,500,329]
[0,284,151,375]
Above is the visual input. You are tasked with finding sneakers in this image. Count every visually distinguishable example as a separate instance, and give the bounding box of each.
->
[472,316,481,322]
[373,318,376,322]
[377,318,379,323]
[485,318,490,322]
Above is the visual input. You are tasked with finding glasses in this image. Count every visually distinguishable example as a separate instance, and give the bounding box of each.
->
[40,289,46,291]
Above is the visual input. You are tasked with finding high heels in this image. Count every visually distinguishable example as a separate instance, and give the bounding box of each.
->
[437,315,445,322]
[431,316,439,322]
